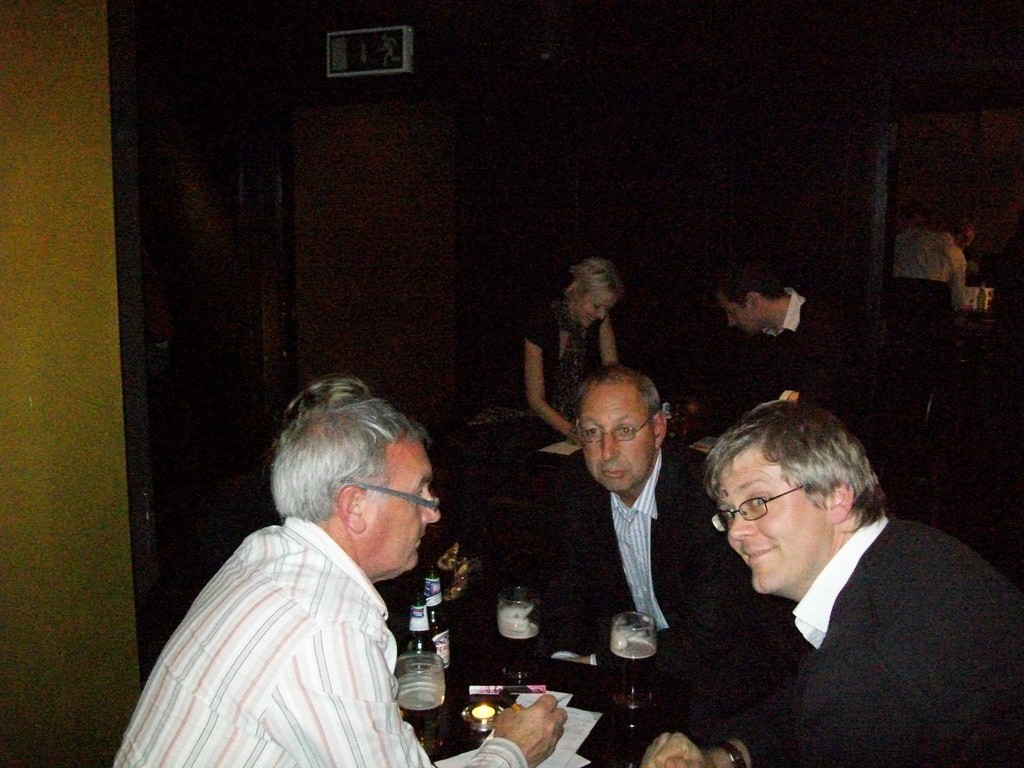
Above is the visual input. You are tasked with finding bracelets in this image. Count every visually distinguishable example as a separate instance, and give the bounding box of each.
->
[705,740,747,768]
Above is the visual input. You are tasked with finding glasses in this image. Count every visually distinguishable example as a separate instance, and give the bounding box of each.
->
[328,479,440,512]
[579,407,661,443]
[712,486,803,532]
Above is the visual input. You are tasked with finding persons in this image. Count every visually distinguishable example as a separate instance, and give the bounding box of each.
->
[111,201,1024,768]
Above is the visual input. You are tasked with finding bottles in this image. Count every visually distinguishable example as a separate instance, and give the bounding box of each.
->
[402,592,438,657]
[422,565,451,683]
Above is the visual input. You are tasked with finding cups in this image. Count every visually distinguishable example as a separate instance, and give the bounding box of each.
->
[609,612,658,708]
[394,651,447,755]
[496,586,543,679]
